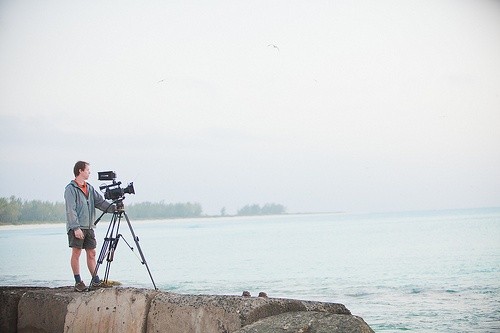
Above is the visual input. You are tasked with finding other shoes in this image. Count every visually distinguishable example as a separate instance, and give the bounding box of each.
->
[87,280,105,290]
[74,277,88,293]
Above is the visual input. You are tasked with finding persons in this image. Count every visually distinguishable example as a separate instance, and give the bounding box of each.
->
[63,160,122,292]
